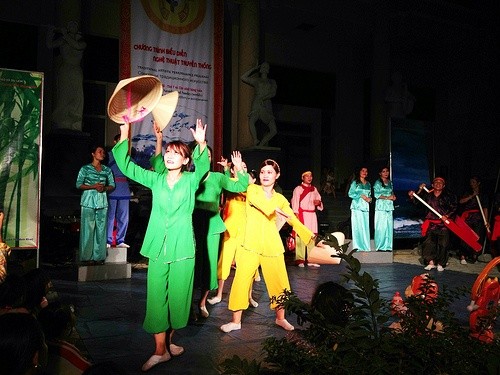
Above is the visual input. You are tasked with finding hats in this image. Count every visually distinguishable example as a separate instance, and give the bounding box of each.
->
[151,90,179,131]
[107,75,163,124]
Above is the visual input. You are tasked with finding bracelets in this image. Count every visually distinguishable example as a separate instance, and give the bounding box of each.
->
[360,195,363,198]
[384,196,388,199]
[369,197,372,202]
[89,186,92,188]
[106,186,109,190]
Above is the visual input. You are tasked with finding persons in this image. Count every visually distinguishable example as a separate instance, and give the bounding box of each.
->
[112,116,210,371]
[409,175,500,271]
[292,170,324,267]
[103,134,134,248]
[348,167,372,252]
[152,121,316,331]
[373,167,396,251]
[76,146,115,265]
[0,211,50,375]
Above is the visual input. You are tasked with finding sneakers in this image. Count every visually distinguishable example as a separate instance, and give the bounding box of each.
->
[200,306,210,317]
[249,298,259,307]
[276,317,293,331]
[168,341,184,357]
[116,243,131,248]
[207,297,222,304]
[143,351,171,369]
[424,264,435,270]
[219,321,243,331]
[460,259,468,264]
[437,264,444,271]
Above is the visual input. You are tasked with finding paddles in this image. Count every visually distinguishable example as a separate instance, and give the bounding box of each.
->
[413,192,482,252]
[423,186,480,242]
[475,193,492,239]
